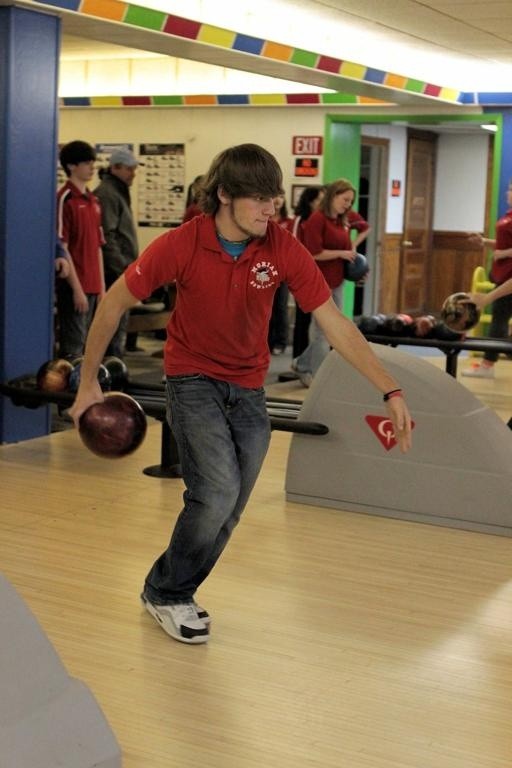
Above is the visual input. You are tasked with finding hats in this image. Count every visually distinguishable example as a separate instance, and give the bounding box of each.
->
[107,147,146,169]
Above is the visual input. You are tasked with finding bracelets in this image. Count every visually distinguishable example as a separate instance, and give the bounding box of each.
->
[381,386,403,398]
[382,392,401,401]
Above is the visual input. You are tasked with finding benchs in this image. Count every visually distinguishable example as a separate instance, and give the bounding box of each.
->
[126,300,174,334]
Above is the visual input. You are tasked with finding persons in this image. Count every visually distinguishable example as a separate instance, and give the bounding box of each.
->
[56,237,68,275]
[460,179,512,380]
[94,147,145,360]
[64,144,413,645]
[269,184,372,392]
[456,277,511,311]
[58,139,108,423]
[182,171,208,226]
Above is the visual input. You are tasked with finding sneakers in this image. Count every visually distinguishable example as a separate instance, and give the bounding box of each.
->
[139,588,212,646]
[290,354,316,389]
[461,362,498,380]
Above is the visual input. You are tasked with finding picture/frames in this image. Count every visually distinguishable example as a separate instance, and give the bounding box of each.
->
[291,184,309,209]
